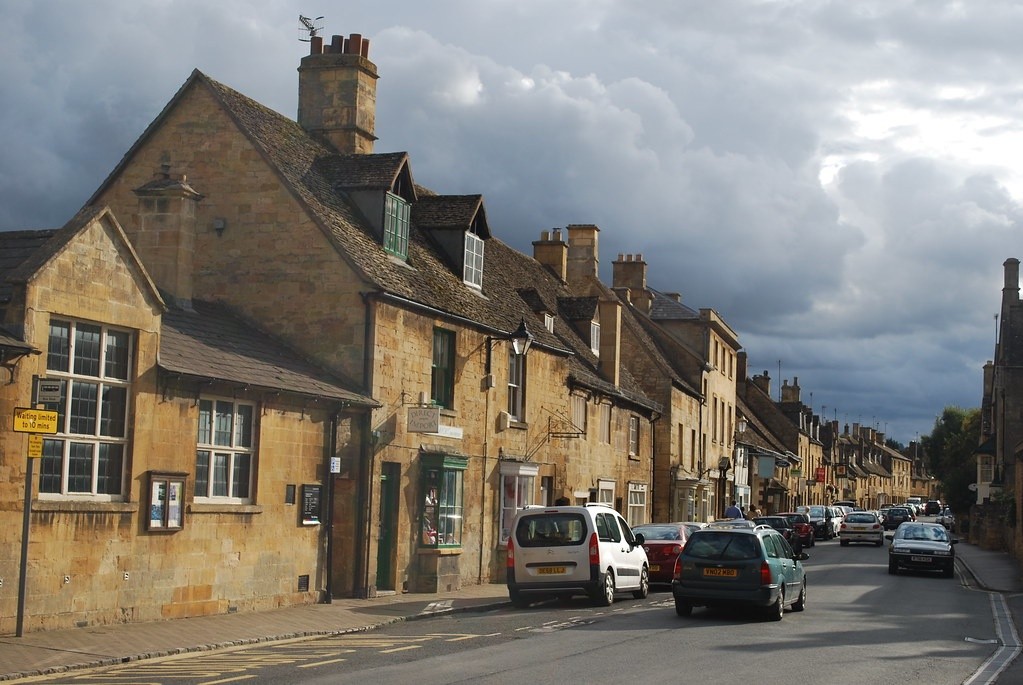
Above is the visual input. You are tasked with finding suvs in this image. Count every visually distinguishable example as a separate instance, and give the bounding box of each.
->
[673,524,810,621]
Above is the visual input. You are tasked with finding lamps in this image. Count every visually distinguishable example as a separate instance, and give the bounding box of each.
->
[486,313,535,375]
[733,415,748,445]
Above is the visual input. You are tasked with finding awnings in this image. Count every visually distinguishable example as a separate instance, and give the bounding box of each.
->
[770,476,791,491]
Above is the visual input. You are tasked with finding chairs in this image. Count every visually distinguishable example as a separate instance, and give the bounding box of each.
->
[535,516,561,539]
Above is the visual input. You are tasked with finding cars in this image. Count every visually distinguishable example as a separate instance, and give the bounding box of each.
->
[925,501,940,516]
[672,522,707,533]
[935,512,944,524]
[866,497,926,531]
[633,524,689,584]
[885,522,959,578]
[708,501,864,556]
[941,508,952,530]
[839,512,884,547]
[506,502,651,608]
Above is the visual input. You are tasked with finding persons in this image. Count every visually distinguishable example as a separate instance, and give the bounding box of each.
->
[803,505,811,524]
[747,504,761,520]
[740,507,748,519]
[725,501,743,519]
